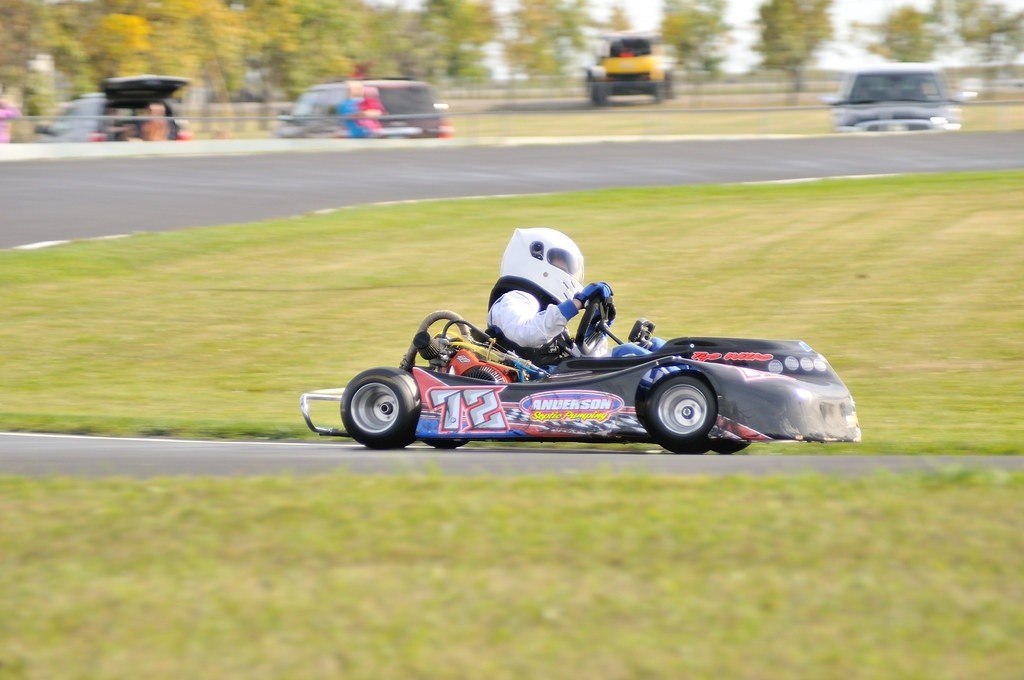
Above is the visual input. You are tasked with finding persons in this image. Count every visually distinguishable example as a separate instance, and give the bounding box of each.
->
[337,81,385,140]
[0,97,21,142]
[488,229,664,381]
[120,103,177,141]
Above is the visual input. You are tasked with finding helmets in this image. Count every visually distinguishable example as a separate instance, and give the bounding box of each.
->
[501,227,586,303]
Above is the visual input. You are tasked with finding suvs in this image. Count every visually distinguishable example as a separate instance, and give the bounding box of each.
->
[581,28,675,103]
[272,76,450,140]
[822,62,978,132]
[34,75,195,145]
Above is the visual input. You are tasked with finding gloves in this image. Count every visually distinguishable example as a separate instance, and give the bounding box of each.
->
[574,282,614,305]
[584,303,615,344]
